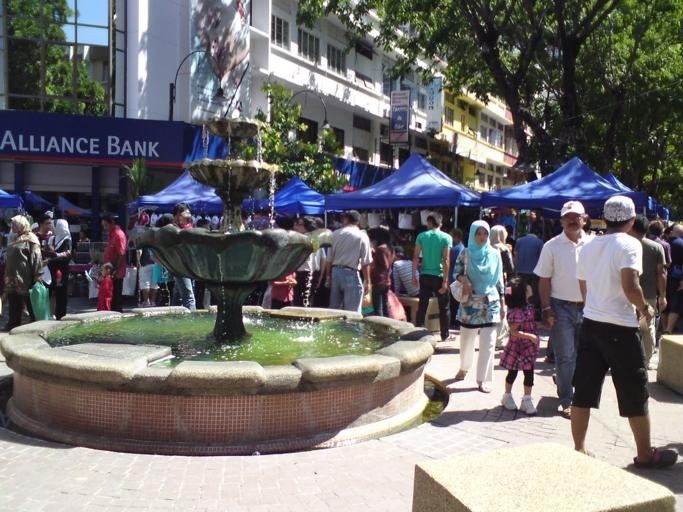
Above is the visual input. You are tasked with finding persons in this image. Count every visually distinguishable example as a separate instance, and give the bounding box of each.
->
[568,192,679,471]
[499,279,541,415]
[452,221,507,394]
[533,199,598,423]
[0,198,682,361]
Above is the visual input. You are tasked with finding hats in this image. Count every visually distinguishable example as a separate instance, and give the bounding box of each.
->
[561,201,584,216]
[603,196,636,222]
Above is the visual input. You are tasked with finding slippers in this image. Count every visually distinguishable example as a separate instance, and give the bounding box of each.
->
[633,447,678,468]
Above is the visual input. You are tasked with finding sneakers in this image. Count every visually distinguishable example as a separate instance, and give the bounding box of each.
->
[455,369,571,419]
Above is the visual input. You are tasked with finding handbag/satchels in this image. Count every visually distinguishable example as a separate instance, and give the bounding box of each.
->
[449,277,469,304]
[29,282,51,321]
